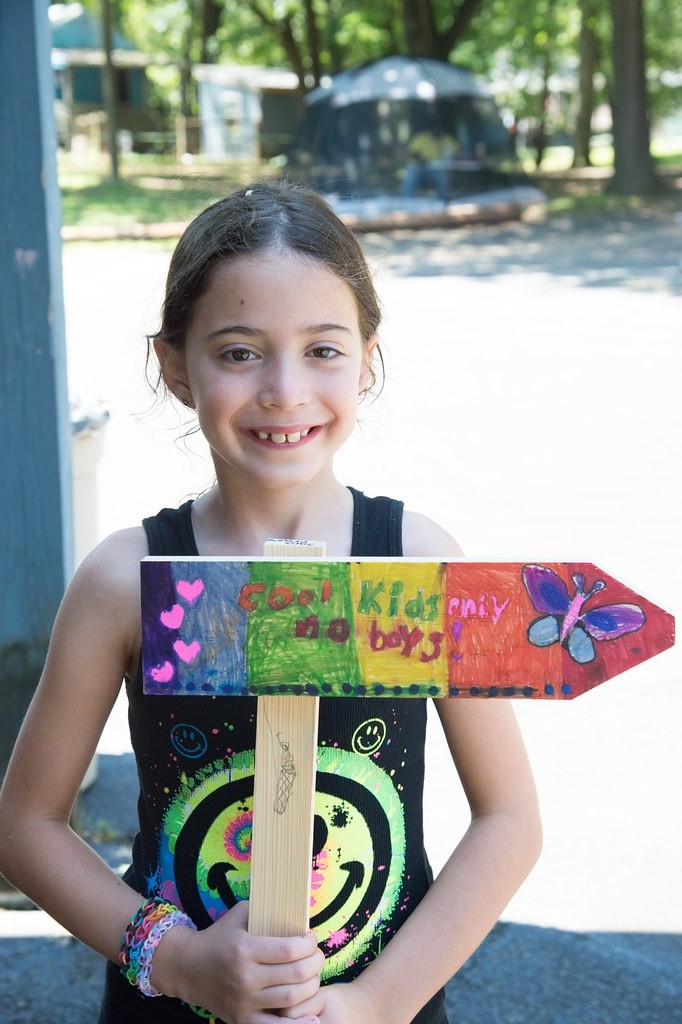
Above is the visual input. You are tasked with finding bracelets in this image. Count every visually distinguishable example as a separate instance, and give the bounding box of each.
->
[119,898,198,997]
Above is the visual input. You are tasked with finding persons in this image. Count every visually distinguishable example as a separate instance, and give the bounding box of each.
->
[0,184,544,1024]
